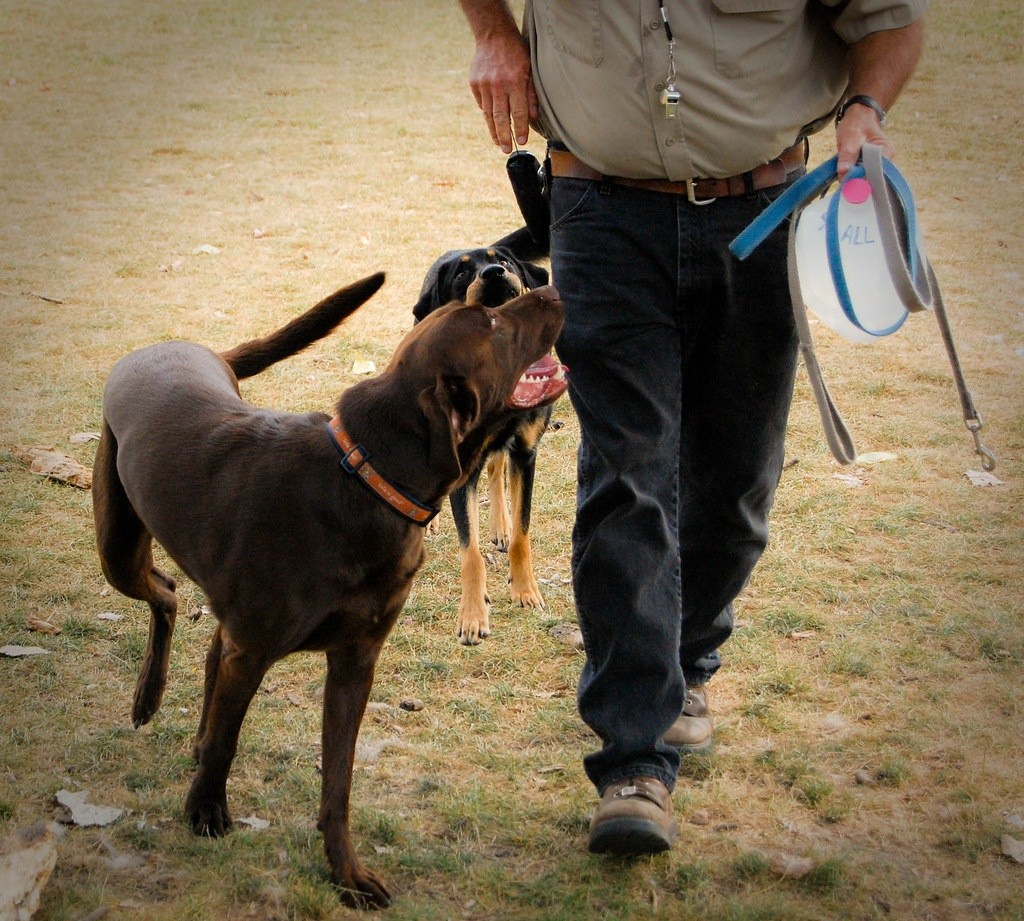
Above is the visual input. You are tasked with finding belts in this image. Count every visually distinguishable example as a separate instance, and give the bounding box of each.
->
[549,137,807,205]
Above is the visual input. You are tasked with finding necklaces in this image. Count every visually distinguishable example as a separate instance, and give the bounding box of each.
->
[656,0,680,119]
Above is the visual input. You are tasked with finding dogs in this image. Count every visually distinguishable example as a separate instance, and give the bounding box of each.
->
[92,225,569,912]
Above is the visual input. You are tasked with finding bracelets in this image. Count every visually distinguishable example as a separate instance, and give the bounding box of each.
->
[834,94,887,127]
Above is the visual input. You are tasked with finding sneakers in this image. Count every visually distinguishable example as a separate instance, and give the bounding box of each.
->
[588,777,680,853]
[659,683,714,757]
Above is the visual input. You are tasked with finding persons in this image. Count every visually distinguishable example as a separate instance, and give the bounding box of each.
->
[459,0,925,851]
